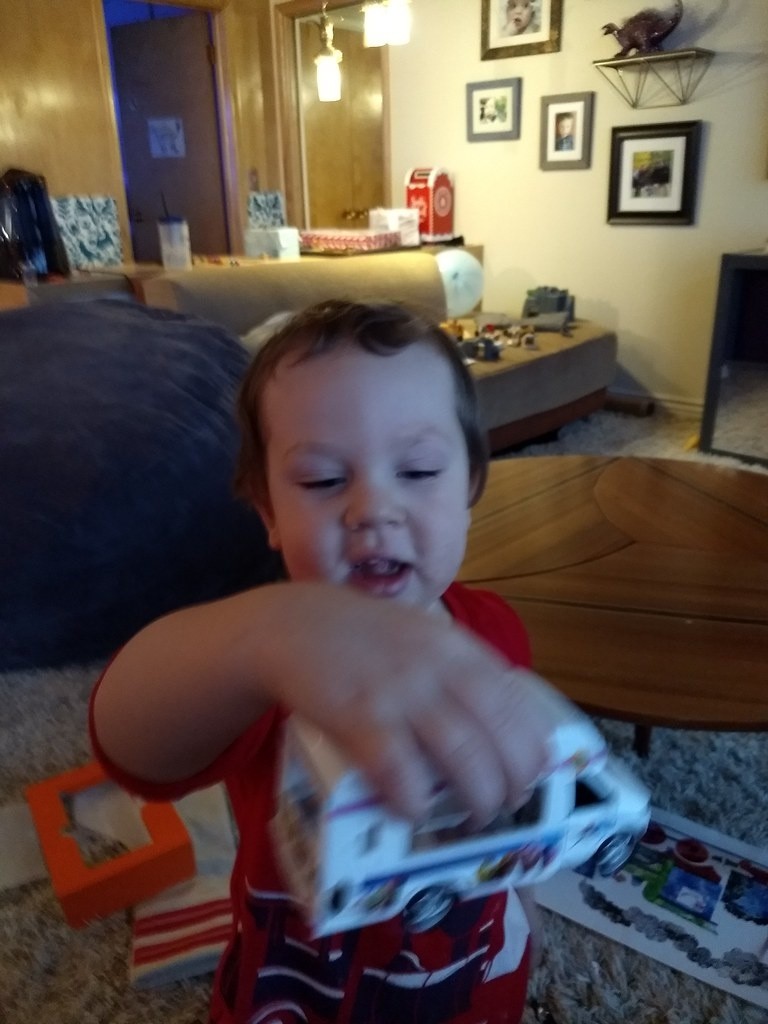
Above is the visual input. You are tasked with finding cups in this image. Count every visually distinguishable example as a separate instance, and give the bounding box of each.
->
[155,215,192,272]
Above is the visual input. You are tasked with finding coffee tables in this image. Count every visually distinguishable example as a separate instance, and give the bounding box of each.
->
[452,453,768,762]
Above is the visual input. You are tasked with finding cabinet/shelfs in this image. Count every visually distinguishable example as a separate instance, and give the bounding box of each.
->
[298,22,392,232]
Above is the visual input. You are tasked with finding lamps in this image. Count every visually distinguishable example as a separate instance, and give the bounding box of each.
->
[304,0,413,102]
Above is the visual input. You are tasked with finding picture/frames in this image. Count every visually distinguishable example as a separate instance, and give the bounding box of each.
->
[465,76,521,142]
[481,0,563,61]
[606,119,703,225]
[538,91,595,170]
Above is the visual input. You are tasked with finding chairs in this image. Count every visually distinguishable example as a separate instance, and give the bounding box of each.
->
[247,190,287,230]
[48,195,124,268]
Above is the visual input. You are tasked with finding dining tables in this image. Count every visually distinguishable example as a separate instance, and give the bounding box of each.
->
[0,255,253,311]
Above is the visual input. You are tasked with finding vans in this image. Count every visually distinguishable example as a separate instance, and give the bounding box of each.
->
[269,691,649,935]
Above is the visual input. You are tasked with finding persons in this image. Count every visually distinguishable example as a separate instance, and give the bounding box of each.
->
[501,0,540,37]
[555,112,575,150]
[3,300,651,1024]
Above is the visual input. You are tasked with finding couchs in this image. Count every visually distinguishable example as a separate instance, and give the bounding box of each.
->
[143,249,619,455]
